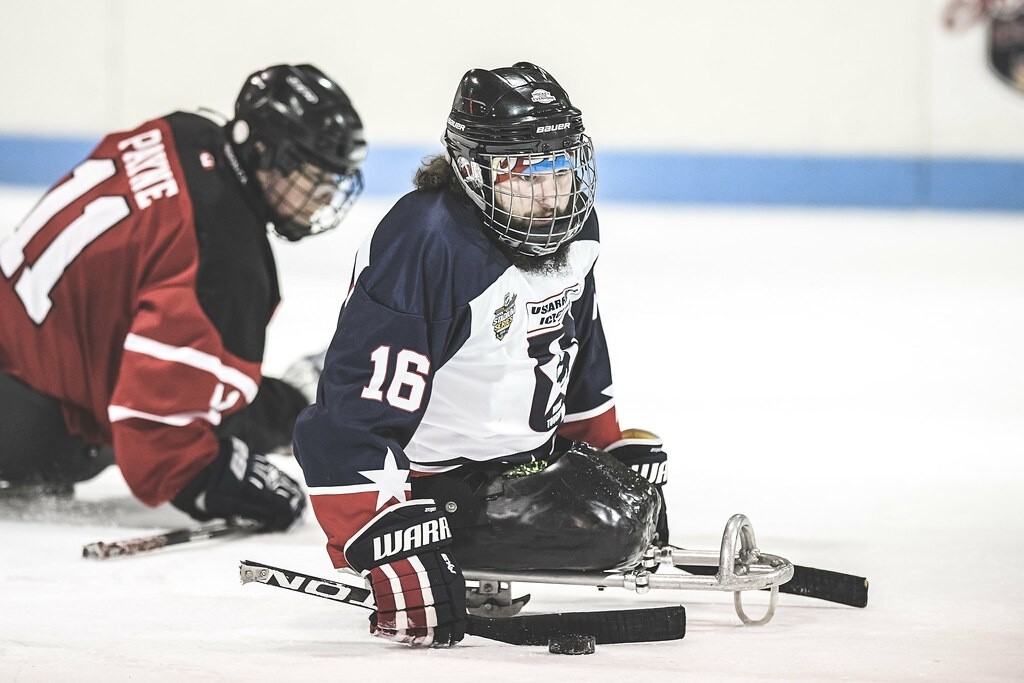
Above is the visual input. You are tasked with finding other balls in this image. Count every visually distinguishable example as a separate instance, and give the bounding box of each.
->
[548,634,596,655]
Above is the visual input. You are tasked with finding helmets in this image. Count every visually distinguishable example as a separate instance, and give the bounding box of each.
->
[231,63,369,245]
[446,58,597,265]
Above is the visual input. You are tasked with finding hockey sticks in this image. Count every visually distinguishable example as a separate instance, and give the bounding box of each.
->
[239,562,688,645]
[657,542,868,609]
[85,516,255,559]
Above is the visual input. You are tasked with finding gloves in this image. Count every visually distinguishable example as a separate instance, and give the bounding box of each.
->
[347,492,470,650]
[173,431,307,535]
[606,427,672,555]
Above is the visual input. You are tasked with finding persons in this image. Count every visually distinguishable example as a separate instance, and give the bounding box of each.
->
[293,62,668,648]
[0,65,367,533]
